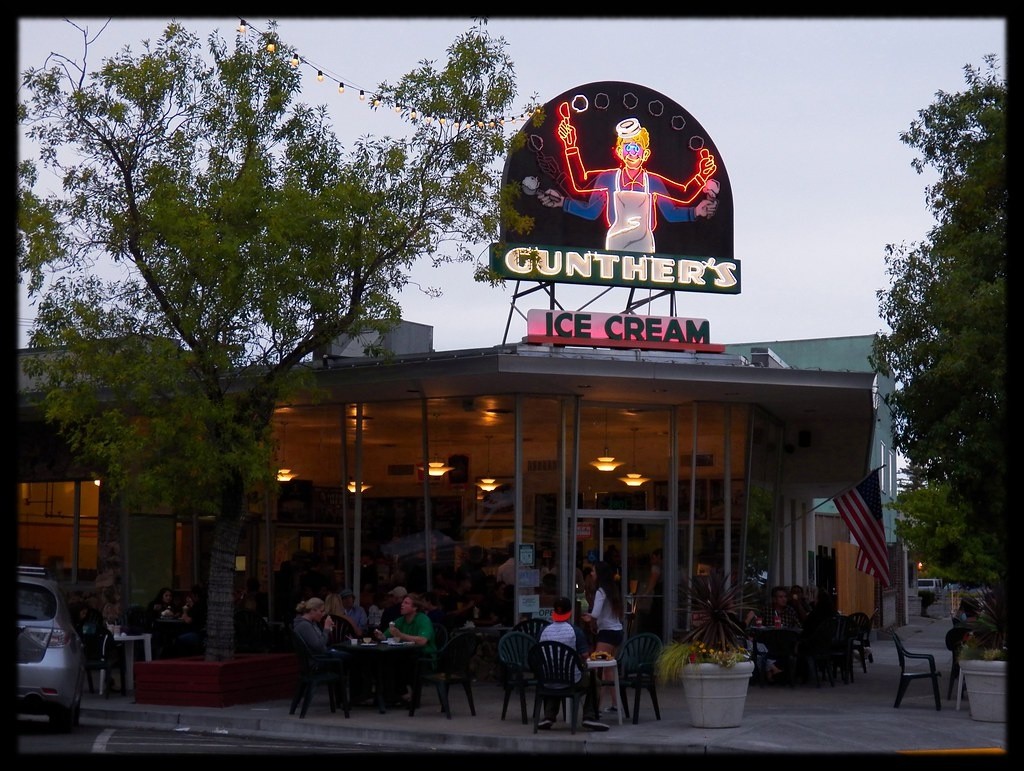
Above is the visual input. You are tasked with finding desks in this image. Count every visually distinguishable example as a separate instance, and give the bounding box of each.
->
[566,656,626,729]
[152,616,190,651]
[100,634,152,695]
[329,639,422,717]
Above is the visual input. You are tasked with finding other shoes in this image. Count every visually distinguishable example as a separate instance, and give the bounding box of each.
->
[583,720,608,730]
[537,717,552,729]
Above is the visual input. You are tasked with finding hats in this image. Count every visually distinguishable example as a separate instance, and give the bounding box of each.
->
[387,586,407,598]
[552,597,572,621]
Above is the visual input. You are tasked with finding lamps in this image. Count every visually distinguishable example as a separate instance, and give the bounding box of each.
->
[474,435,505,492]
[274,421,302,482]
[588,407,627,471]
[418,412,455,476]
[347,443,373,493]
[616,428,652,486]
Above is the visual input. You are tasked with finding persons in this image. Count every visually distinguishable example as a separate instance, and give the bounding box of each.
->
[742,585,831,686]
[497,536,662,729]
[68,575,268,695]
[281,544,495,707]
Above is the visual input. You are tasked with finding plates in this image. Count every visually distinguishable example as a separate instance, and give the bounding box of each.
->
[361,644,377,646]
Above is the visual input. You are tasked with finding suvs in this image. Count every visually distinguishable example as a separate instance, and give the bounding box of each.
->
[16,564,86,732]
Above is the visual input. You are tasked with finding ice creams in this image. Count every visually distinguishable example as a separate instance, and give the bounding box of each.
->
[183,605,188,614]
[389,621,395,629]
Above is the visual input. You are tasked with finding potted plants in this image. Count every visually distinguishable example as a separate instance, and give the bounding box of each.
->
[653,564,761,728]
[954,584,1006,722]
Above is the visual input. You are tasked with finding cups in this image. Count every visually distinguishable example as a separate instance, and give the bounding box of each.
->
[107,626,114,634]
[363,638,371,644]
[756,618,762,628]
[774,617,781,630]
[114,626,121,637]
[473,606,480,618]
[388,638,397,644]
[351,639,357,645]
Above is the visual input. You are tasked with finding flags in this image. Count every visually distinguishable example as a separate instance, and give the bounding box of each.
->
[837,472,891,589]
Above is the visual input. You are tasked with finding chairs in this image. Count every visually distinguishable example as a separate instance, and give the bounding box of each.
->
[53,588,872,736]
[889,626,943,711]
